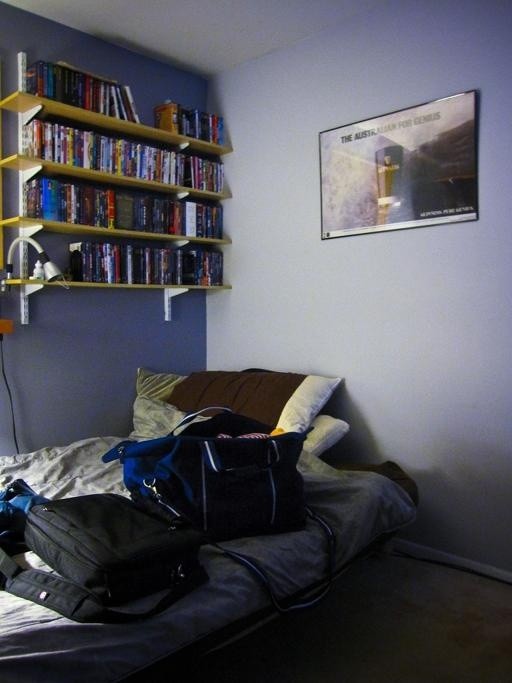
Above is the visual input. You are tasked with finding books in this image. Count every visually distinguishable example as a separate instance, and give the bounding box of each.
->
[26,178,225,240]
[153,103,224,146]
[26,120,224,195]
[69,241,224,285]
[26,60,141,123]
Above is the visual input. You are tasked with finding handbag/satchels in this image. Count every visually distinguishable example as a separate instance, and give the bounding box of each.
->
[0,493,211,625]
[103,404,308,537]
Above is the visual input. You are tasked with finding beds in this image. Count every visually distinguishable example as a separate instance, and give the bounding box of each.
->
[0,423,424,682]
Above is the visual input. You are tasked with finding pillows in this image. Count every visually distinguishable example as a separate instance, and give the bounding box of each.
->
[134,364,354,461]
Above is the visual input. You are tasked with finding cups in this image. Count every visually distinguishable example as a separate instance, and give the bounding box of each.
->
[375,146,402,205]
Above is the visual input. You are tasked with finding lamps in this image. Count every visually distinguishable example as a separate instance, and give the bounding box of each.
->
[1,233,65,292]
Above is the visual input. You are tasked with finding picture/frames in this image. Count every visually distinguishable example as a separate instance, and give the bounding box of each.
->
[315,86,481,243]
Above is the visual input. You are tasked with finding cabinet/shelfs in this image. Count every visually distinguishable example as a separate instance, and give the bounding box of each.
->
[1,52,235,325]
